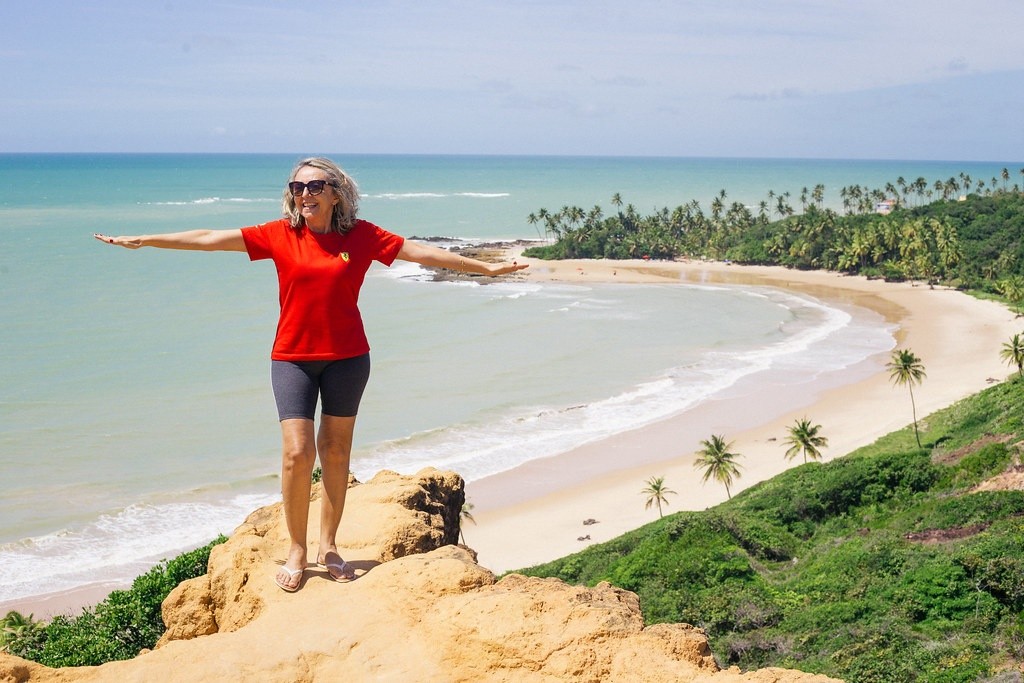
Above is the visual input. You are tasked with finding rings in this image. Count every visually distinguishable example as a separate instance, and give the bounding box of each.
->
[513,261,517,269]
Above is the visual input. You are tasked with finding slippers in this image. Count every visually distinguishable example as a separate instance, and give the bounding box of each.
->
[274,566,303,592]
[317,561,356,583]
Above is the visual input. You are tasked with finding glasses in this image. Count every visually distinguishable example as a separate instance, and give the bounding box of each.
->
[288,180,338,197]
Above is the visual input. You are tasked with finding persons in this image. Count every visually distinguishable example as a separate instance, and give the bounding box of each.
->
[94,157,530,592]
